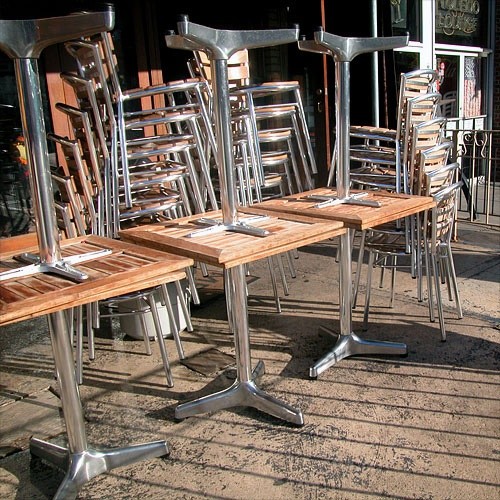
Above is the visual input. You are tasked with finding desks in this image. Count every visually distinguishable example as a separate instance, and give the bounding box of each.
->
[0,12,437,500]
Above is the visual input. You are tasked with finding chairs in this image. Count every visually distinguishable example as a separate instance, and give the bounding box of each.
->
[186,47,318,313]
[48,10,220,388]
[328,69,464,344]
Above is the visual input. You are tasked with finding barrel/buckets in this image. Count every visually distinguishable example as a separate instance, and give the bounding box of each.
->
[119,278,189,339]
